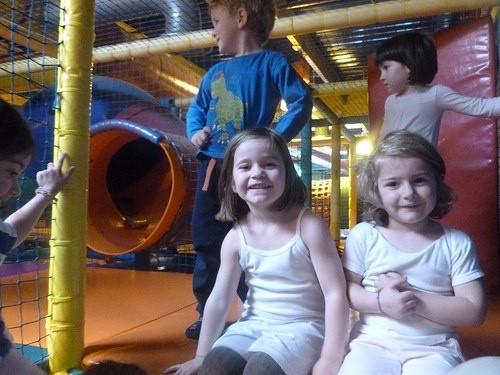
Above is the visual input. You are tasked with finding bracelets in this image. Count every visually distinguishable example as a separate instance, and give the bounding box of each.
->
[377,288,387,315]
[36,187,58,204]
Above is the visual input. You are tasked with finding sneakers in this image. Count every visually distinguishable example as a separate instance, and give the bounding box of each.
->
[185,317,204,338]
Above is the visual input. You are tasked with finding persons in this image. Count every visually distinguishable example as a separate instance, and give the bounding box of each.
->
[337,129,487,375]
[0,94,76,375]
[373,32,500,148]
[165,127,348,375]
[445,357,500,375]
[186,0,313,337]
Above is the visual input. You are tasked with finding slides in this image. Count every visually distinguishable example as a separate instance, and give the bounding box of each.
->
[17,75,203,257]
